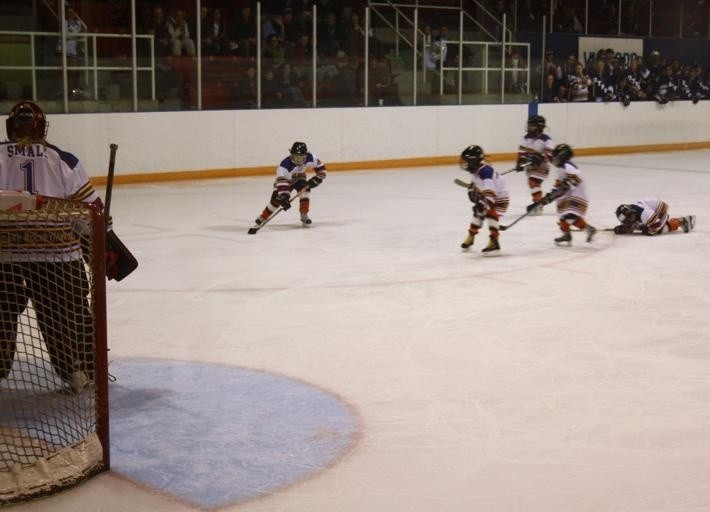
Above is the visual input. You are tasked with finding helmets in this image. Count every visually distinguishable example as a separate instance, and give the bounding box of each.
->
[525,115,545,138]
[459,145,485,174]
[5,102,49,142]
[552,144,573,167]
[616,205,637,225]
[289,142,308,165]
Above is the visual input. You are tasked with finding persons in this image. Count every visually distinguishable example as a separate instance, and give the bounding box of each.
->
[0,100,113,396]
[481,1,708,103]
[98,2,399,105]
[399,23,474,95]
[247,142,328,235]
[0,0,94,100]
[454,115,696,251]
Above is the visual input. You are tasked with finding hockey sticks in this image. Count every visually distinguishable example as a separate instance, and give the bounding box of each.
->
[557,222,613,231]
[499,204,540,230]
[455,161,532,189]
[248,186,310,235]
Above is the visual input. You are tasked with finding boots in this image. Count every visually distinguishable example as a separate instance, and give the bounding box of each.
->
[461,230,478,248]
[482,235,500,252]
[301,212,311,224]
[256,217,264,225]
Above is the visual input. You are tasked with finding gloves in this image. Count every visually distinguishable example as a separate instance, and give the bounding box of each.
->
[473,202,489,218]
[468,191,484,203]
[531,153,544,168]
[517,159,529,171]
[306,176,322,192]
[279,193,291,210]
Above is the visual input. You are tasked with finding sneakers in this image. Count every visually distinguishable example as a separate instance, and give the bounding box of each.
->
[680,215,694,233]
[587,226,595,242]
[555,235,571,242]
